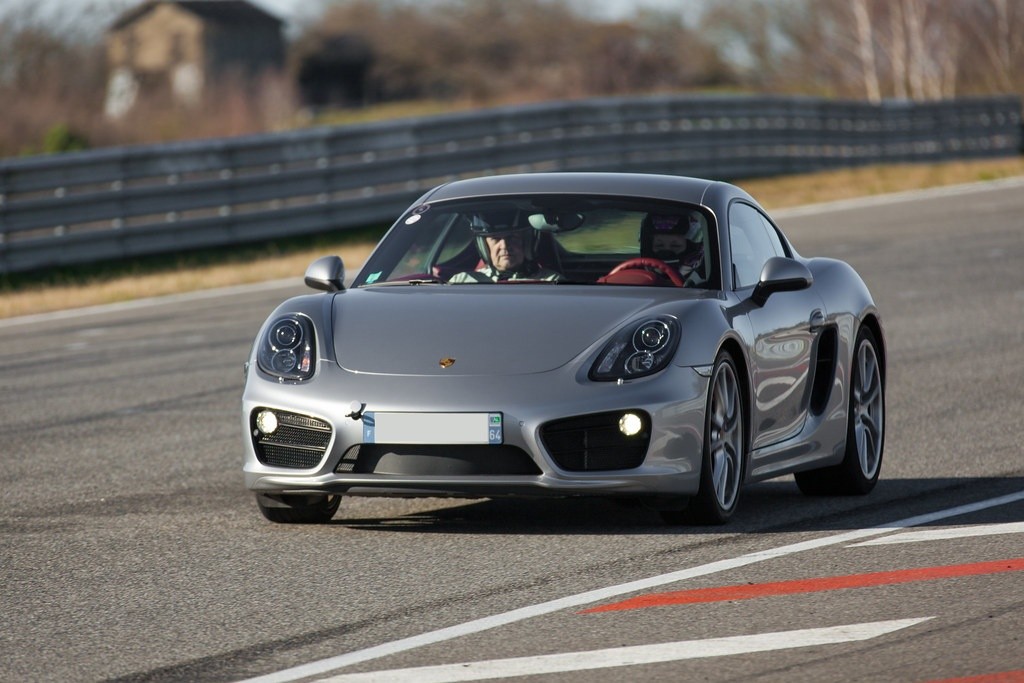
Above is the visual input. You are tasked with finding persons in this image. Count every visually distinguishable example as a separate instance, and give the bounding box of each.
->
[445,209,564,284]
[640,211,705,284]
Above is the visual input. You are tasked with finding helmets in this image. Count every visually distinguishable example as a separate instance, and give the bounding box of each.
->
[469,205,542,266]
[640,204,705,276]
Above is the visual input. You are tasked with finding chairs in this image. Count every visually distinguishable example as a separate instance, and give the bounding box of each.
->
[475,231,561,272]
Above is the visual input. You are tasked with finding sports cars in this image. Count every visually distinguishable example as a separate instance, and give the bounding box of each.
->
[242,174,889,531]
